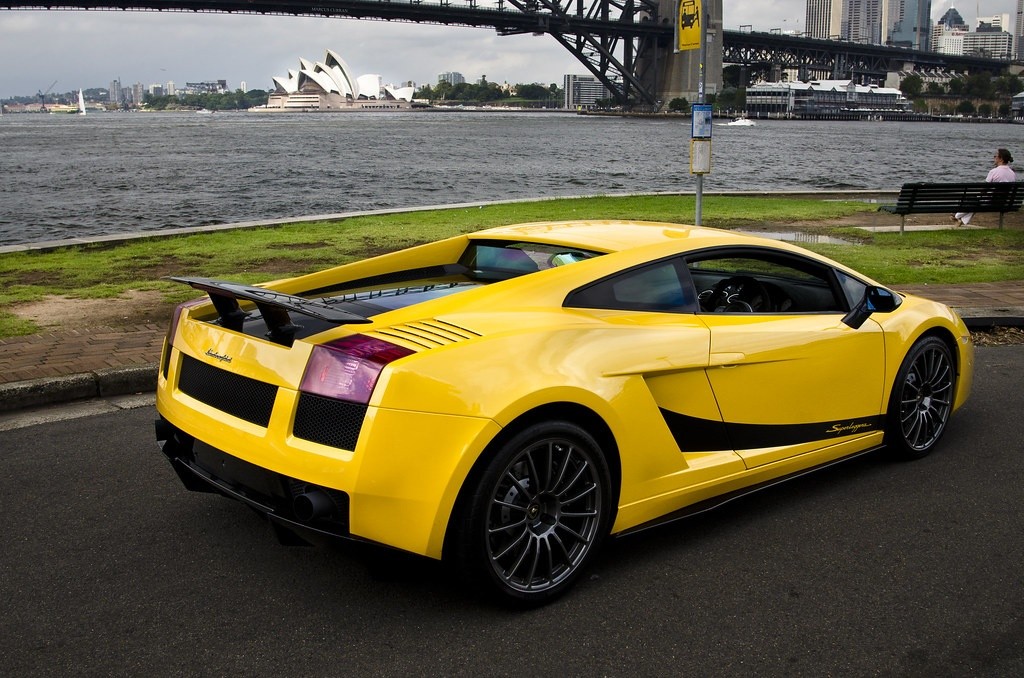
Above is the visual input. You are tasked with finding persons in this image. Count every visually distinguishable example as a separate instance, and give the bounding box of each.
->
[951,148,1016,227]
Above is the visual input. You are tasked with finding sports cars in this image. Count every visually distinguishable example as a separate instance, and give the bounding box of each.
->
[155,220,975,612]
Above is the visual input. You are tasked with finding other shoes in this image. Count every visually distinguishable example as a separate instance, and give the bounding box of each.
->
[949,214,957,223]
[956,219,963,227]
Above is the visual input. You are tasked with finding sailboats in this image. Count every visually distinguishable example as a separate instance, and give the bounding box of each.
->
[76,89,87,115]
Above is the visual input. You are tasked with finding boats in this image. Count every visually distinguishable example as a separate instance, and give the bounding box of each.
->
[727,115,756,127]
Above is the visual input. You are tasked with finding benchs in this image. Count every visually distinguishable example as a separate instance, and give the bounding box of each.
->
[877,182,1024,235]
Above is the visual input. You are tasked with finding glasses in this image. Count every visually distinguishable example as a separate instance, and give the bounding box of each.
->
[993,155,999,158]
